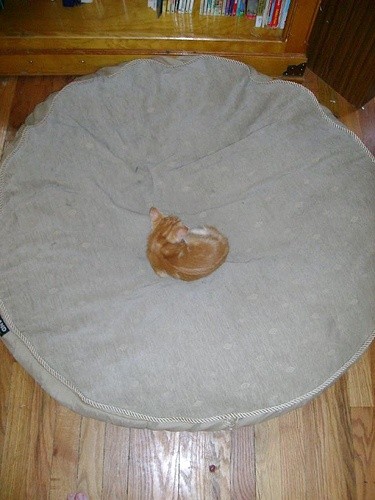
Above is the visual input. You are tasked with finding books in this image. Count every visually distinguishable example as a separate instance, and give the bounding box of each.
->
[146,0,194,18]
[255,1,290,29]
[199,0,257,20]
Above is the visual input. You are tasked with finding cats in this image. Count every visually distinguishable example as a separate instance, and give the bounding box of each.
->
[146,206,230,281]
